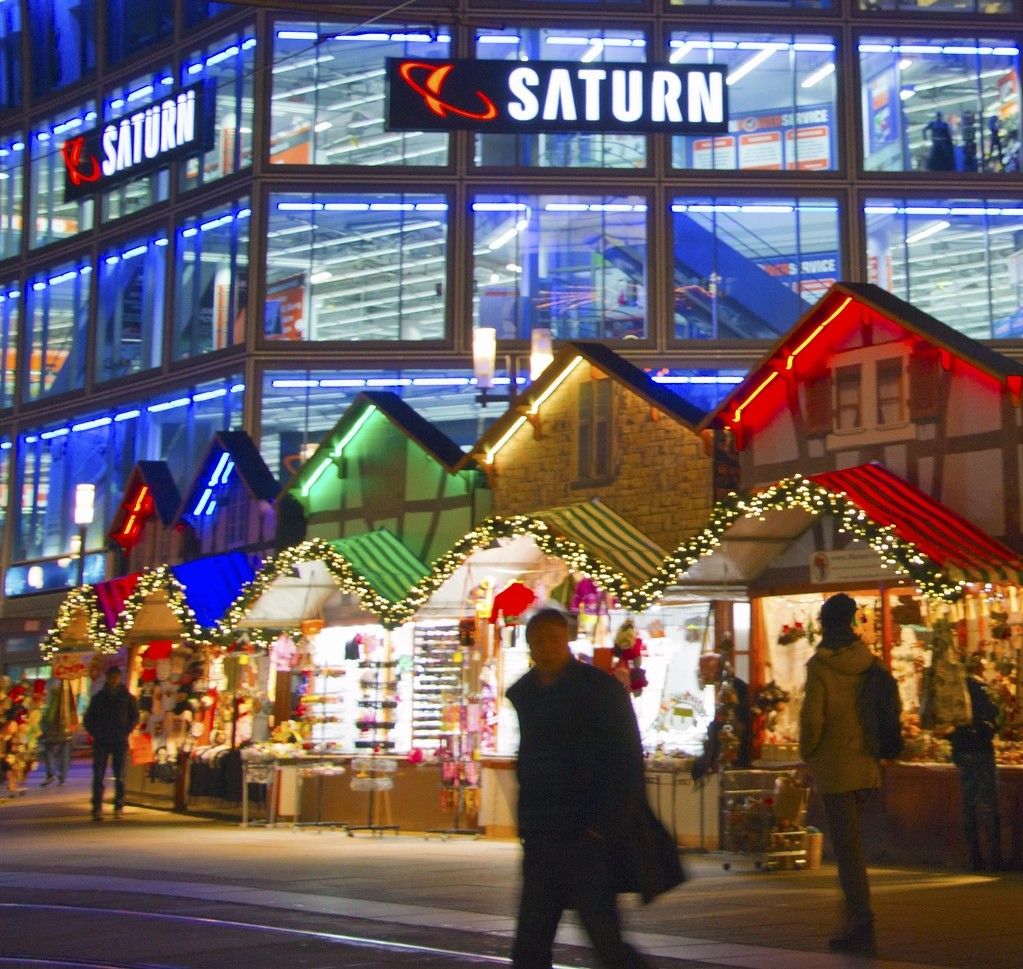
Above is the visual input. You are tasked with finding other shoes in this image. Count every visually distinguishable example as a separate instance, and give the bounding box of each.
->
[827,919,888,955]
[58,778,66,787]
[91,809,107,821]
[41,775,58,786]
[113,808,124,820]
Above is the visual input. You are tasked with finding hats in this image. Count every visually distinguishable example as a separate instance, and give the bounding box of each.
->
[50,680,64,690]
[104,665,123,675]
[816,594,858,623]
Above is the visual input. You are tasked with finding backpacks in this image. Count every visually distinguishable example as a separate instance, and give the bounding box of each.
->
[863,668,907,761]
[588,786,686,898]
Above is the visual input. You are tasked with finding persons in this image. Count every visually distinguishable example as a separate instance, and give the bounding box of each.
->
[944,656,1012,873]
[39,681,71,788]
[985,116,1005,172]
[800,592,891,956]
[83,666,140,820]
[923,111,955,170]
[504,607,687,969]
